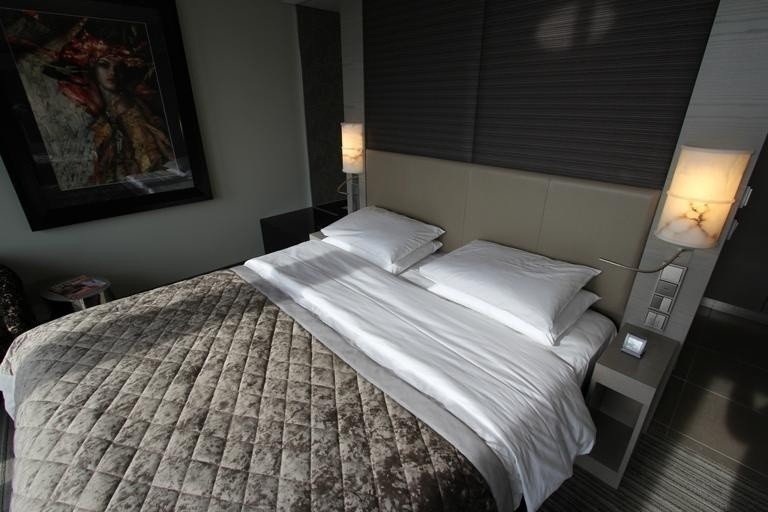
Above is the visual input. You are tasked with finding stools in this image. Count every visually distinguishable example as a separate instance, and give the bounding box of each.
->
[35,273,114,311]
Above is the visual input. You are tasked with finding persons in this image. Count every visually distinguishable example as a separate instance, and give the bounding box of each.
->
[59,22,174,186]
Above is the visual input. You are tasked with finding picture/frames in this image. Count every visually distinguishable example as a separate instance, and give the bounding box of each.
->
[0,0,214,234]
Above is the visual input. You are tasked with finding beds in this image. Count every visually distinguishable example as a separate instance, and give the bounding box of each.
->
[1,147,663,512]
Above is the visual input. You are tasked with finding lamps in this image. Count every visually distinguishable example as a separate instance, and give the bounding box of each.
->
[652,142,754,253]
[340,122,367,179]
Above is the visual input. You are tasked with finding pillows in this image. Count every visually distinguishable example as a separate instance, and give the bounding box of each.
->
[316,205,446,276]
[422,230,600,336]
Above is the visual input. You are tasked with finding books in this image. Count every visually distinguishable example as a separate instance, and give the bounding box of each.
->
[126,168,194,194]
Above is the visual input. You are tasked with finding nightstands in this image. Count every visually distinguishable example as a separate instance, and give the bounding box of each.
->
[572,332,680,491]
[260,201,342,254]
